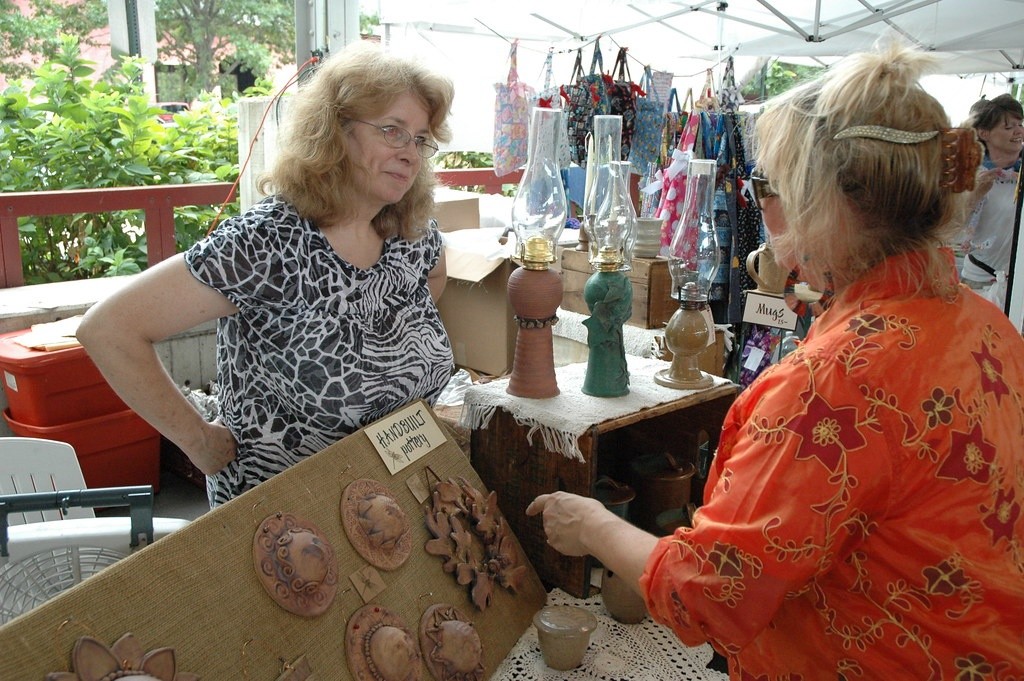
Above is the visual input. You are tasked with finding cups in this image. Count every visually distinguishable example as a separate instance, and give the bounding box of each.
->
[599,565,646,625]
[647,450,695,505]
[533,605,598,670]
[595,473,636,526]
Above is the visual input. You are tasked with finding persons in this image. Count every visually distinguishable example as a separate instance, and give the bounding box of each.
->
[526,38,1024,681]
[75,39,458,510]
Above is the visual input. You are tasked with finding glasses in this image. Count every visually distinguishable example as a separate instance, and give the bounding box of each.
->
[749,163,780,209]
[343,117,439,158]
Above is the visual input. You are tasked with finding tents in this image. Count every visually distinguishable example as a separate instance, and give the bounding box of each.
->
[378,0,1023,154]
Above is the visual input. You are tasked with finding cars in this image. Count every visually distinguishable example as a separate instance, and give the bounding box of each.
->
[155,102,188,124]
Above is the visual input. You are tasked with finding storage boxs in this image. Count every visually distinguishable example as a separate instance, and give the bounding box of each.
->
[429,189,562,382]
[0,313,167,494]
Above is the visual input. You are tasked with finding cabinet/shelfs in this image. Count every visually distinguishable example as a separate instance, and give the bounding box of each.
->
[464,355,747,599]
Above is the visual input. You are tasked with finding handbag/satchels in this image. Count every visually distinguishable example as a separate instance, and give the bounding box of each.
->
[493,37,771,326]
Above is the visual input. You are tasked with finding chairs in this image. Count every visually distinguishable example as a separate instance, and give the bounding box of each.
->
[0,434,109,621]
